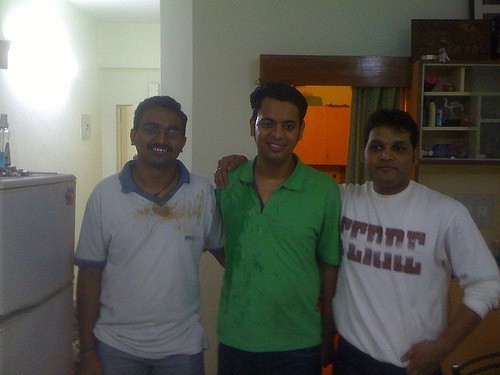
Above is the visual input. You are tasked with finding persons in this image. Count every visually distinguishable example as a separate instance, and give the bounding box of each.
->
[209,109,500,375]
[73,95,230,375]
[214,78,342,375]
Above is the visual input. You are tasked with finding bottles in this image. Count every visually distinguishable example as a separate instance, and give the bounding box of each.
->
[0,113,12,176]
[422,96,465,127]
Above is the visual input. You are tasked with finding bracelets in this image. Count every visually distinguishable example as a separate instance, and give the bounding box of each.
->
[79,347,98,356]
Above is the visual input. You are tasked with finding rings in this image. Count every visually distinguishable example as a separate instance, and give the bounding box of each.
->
[217,167,221,170]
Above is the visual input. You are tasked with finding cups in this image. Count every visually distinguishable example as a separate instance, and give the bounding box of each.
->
[432,144,450,158]
[452,143,468,158]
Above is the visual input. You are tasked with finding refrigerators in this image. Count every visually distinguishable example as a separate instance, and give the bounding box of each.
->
[0,174,77,375]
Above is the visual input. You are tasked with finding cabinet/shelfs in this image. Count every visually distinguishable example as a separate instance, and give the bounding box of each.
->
[417,59,500,162]
